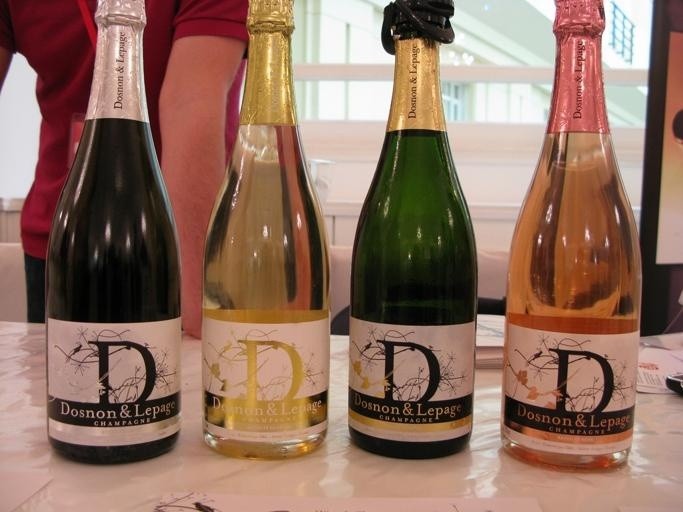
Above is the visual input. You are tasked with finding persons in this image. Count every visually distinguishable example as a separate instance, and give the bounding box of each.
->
[0,2,250,341]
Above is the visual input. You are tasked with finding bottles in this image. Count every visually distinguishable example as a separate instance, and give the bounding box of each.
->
[344,0,477,459]
[495,1,640,472]
[46,0,180,463]
[199,0,332,462]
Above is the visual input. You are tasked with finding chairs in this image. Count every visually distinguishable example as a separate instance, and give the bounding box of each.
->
[331,295,506,336]
